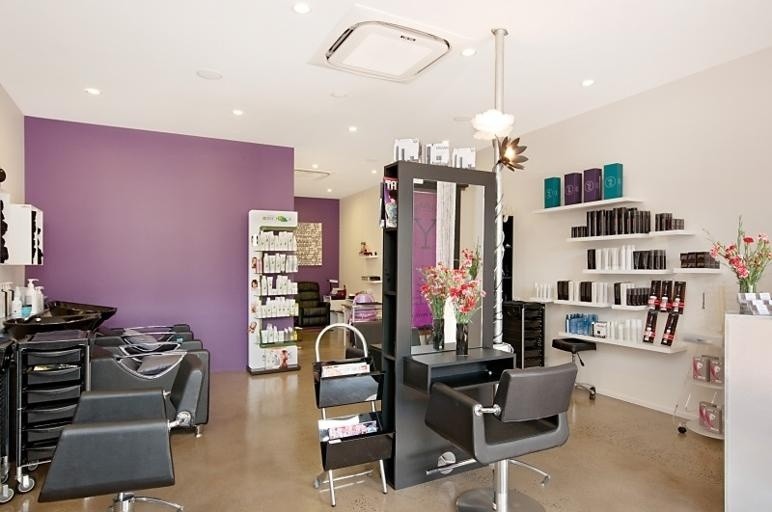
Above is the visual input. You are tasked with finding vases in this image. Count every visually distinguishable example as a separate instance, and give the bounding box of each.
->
[455,323,470,357]
[733,292,771,314]
[430,318,446,351]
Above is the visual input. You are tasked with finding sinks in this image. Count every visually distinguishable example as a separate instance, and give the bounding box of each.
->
[3,313,101,340]
[47,300,117,328]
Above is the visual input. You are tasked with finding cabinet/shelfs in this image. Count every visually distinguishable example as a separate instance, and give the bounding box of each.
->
[528,196,721,358]
[0,170,45,268]
[376,161,503,490]
[248,209,302,374]
[361,254,381,287]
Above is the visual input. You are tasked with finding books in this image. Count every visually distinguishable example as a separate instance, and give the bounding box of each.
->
[321,362,371,379]
[327,419,379,440]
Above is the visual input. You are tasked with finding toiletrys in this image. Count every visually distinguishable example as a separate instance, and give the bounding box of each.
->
[532,207,720,346]
[259,230,300,343]
[0,278,46,330]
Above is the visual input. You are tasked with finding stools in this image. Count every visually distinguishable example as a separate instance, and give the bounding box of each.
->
[552,337,597,400]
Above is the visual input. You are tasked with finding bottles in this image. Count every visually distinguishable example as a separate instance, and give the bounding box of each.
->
[564,312,597,336]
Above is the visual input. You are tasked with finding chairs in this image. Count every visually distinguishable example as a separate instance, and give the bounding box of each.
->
[39,354,205,512]
[292,281,330,326]
[420,362,580,512]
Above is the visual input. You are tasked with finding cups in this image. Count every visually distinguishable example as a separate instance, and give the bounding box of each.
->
[361,241,366,253]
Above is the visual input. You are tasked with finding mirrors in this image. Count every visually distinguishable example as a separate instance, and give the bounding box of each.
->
[411,176,486,355]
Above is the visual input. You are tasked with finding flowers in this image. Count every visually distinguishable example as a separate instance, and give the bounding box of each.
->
[449,245,486,319]
[416,260,450,317]
[695,210,771,288]
